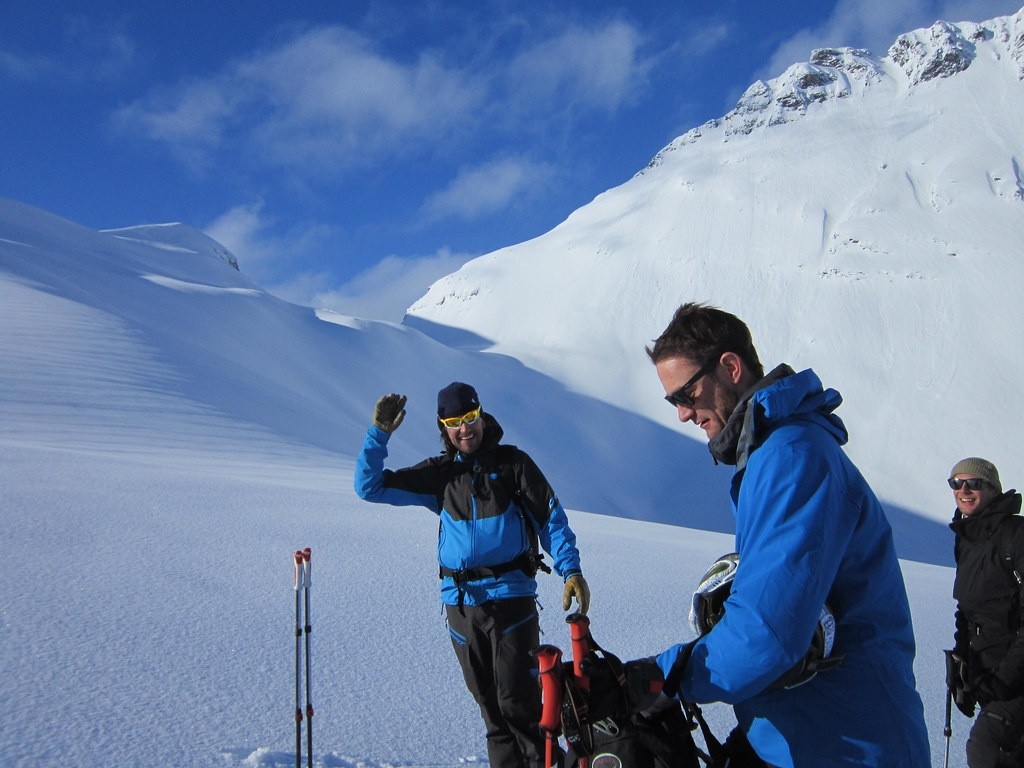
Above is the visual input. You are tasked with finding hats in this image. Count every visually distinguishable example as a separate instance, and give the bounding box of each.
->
[949,457,1002,491]
[436,381,480,419]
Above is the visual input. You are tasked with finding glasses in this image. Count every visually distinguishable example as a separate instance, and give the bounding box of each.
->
[948,478,995,490]
[665,351,725,407]
[437,405,481,429]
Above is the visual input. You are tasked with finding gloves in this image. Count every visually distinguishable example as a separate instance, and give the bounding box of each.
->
[373,393,407,433]
[579,655,664,720]
[563,575,590,615]
[952,655,978,717]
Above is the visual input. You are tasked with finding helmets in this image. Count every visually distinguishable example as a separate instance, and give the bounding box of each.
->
[688,552,840,696]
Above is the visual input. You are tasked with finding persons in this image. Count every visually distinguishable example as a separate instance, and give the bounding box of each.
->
[947,458,1024,768]
[616,304,932,768]
[354,382,590,768]
